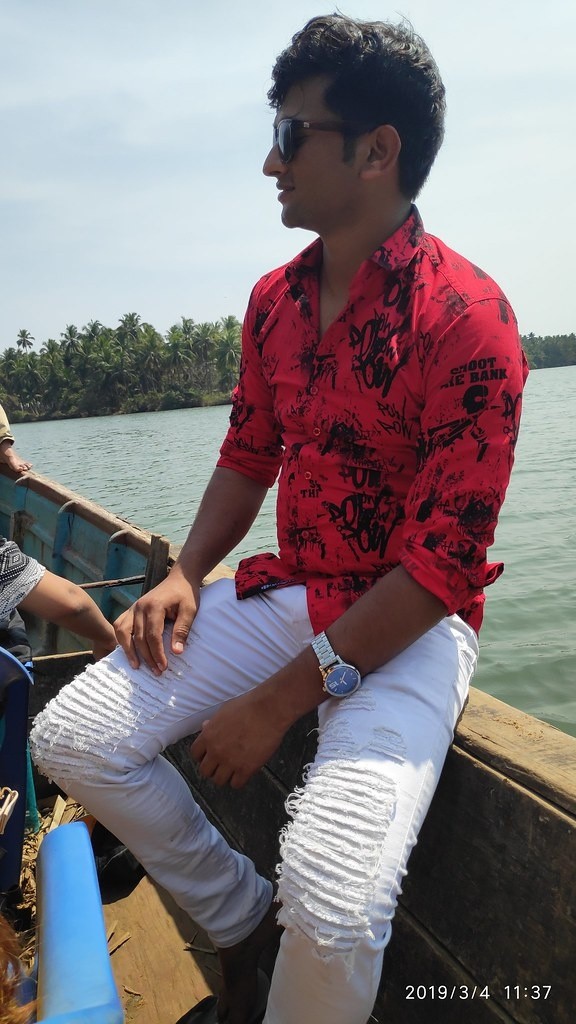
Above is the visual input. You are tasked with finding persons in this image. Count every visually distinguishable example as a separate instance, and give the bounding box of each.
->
[29,13,530,1024]
[0,403,34,473]
[0,532,121,687]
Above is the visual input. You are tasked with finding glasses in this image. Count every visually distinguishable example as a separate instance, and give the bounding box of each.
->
[273,119,380,164]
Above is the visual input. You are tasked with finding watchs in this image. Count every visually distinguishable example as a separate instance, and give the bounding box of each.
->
[309,629,362,699]
